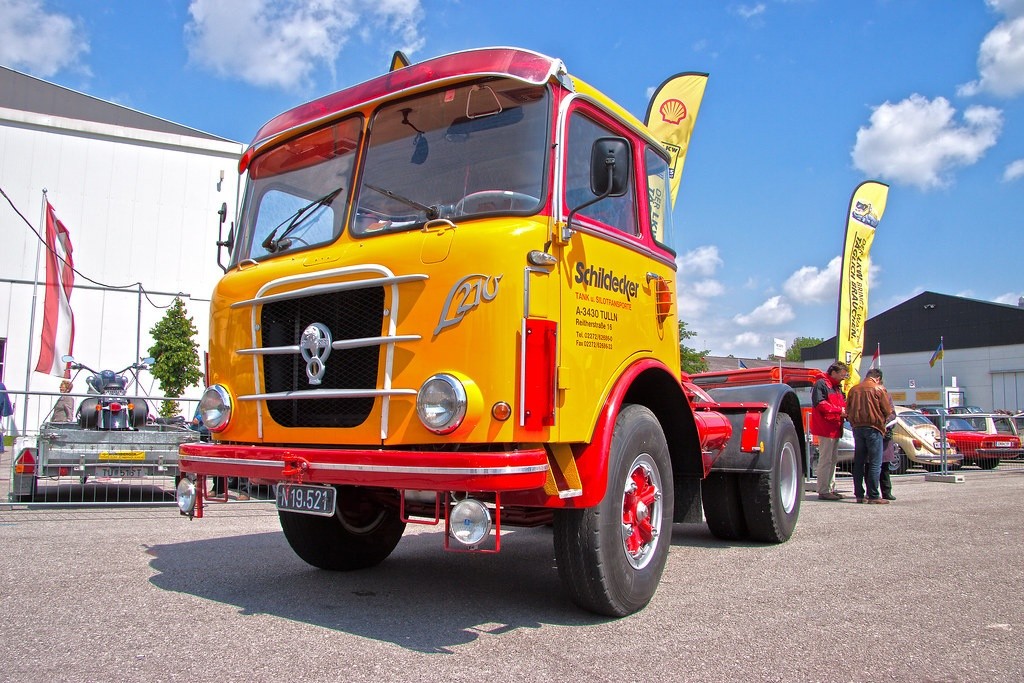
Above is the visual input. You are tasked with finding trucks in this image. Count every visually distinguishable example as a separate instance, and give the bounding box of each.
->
[172,48,809,617]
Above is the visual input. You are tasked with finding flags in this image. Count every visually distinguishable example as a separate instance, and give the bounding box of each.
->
[868,347,879,370]
[929,341,943,368]
[739,360,749,370]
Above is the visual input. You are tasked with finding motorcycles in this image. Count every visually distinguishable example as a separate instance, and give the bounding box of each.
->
[61,355,158,433]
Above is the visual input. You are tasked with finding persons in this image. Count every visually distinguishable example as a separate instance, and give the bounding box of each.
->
[51,381,74,422]
[191,401,225,498]
[0,383,12,452]
[812,361,849,500]
[846,369,896,503]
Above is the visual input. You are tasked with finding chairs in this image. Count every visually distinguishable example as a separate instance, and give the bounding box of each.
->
[566,187,611,225]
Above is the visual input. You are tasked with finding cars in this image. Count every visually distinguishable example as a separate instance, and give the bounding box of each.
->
[835,404,1021,474]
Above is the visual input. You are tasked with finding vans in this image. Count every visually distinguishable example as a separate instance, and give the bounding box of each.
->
[687,367,822,476]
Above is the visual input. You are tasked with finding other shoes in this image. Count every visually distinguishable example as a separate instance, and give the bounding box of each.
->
[857,498,889,504]
[883,495,896,500]
[818,492,845,500]
